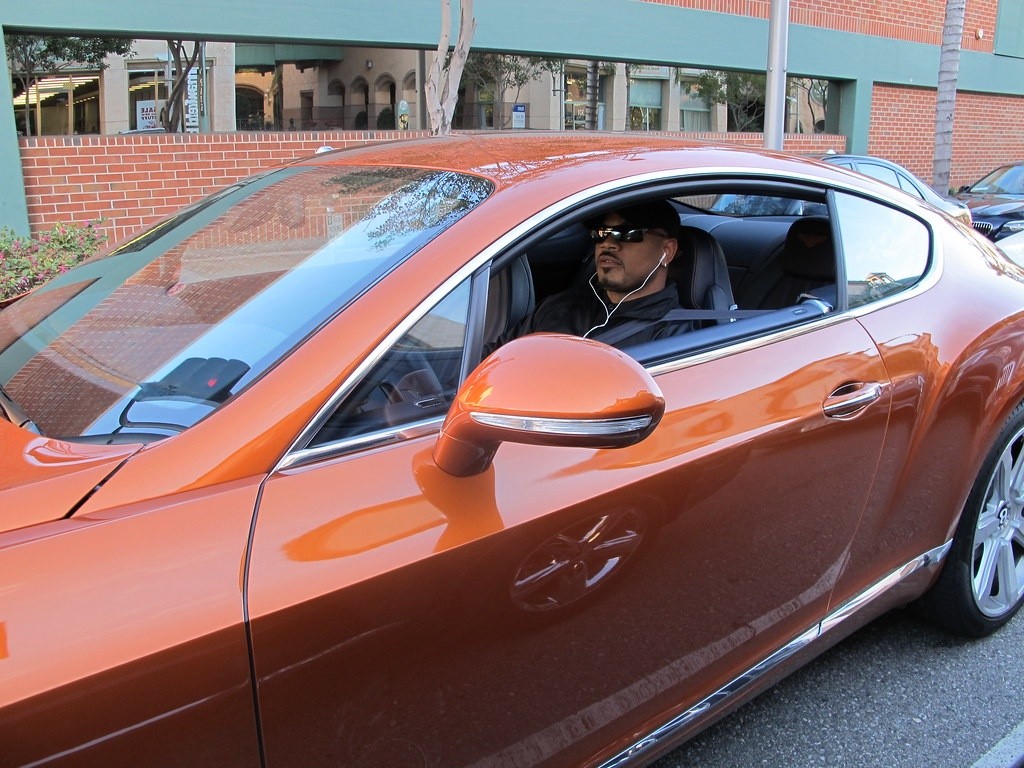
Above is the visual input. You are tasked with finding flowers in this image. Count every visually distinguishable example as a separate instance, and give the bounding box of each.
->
[0,216,109,303]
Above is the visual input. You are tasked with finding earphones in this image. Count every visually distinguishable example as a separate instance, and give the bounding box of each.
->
[660,251,666,264]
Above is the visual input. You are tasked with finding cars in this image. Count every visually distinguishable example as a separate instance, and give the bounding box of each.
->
[954,161,1024,242]
[0,130,1024,768]
[707,149,973,229]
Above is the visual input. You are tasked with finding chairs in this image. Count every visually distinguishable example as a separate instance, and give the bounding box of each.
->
[669,224,739,331]
[776,215,837,309]
[485,256,535,346]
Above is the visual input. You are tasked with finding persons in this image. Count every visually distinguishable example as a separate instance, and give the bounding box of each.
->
[440,197,697,390]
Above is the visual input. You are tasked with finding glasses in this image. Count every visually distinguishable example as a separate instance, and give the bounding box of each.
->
[587,220,667,243]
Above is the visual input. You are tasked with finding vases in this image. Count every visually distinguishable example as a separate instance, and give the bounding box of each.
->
[0,280,47,309]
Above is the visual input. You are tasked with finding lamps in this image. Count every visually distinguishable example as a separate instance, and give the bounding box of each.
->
[366,60,372,69]
